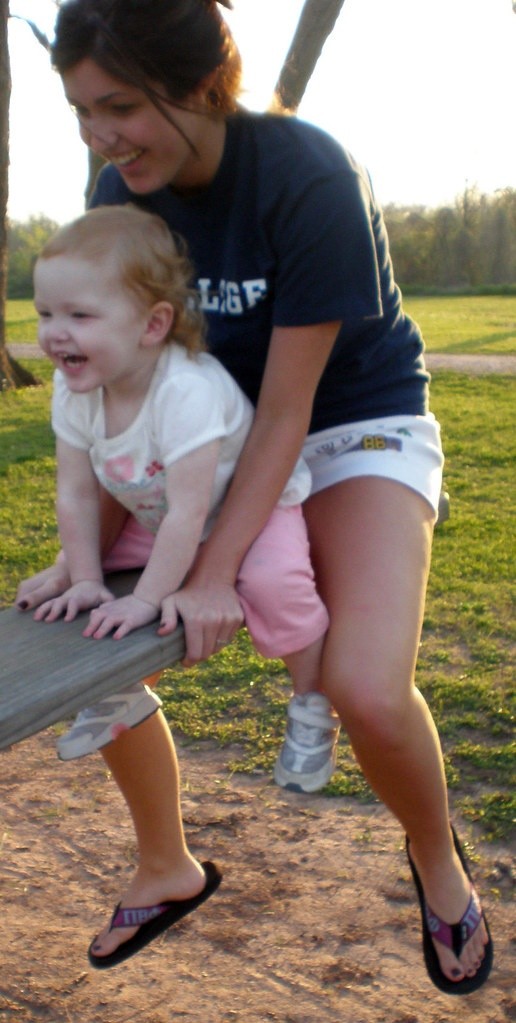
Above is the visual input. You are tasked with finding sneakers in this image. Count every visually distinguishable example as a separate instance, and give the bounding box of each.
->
[56,678,162,761]
[273,690,341,793]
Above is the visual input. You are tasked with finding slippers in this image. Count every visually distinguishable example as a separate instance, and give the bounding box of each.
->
[405,823,493,996]
[88,860,224,969]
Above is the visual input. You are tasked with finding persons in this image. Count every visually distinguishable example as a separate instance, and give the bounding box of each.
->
[33,206,341,794]
[15,0,494,994]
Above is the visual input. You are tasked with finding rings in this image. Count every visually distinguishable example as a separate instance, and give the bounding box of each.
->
[217,640,230,647]
